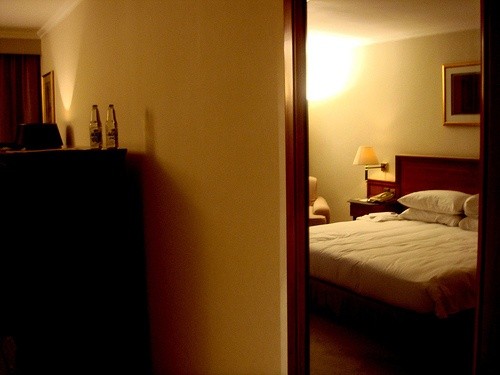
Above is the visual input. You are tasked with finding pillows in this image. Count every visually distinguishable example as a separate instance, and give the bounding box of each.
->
[459,217,478,232]
[463,194,479,217]
[397,190,471,216]
[398,208,460,227]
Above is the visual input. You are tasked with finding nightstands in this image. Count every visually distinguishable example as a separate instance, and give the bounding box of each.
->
[348,200,388,220]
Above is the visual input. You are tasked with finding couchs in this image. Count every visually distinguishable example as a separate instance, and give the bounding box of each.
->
[309,176,330,226]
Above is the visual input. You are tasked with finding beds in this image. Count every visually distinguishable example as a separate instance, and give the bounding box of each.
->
[308,155,479,354]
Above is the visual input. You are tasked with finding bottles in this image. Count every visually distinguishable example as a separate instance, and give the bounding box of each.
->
[105,105,119,150]
[89,104,103,149]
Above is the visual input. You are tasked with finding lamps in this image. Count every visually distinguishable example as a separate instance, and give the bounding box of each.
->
[352,146,389,180]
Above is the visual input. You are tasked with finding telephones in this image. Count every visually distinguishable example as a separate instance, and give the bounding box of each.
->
[370,191,394,202]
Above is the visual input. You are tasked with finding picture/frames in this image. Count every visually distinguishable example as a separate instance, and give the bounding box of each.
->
[41,70,55,123]
[441,62,481,128]
